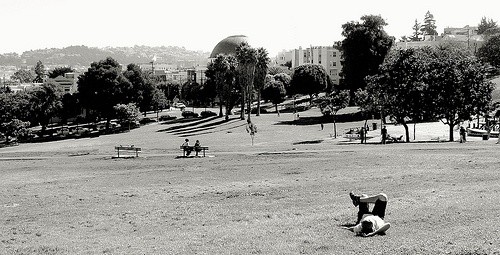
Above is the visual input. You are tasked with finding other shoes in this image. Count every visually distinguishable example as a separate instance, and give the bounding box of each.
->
[349,192,360,207]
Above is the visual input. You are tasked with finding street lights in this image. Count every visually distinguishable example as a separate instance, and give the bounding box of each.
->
[150,62,153,71]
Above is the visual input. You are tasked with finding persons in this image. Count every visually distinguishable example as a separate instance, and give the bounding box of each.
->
[460,127,465,138]
[182,139,191,156]
[195,140,201,156]
[360,127,364,144]
[382,126,387,144]
[321,123,323,130]
[349,191,390,236]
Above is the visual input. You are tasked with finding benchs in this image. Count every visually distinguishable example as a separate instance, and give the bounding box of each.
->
[397,136,403,142]
[180,146,209,157]
[115,147,142,157]
[346,128,360,138]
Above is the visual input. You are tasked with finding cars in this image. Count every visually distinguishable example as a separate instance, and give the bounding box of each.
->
[176,103,186,108]
